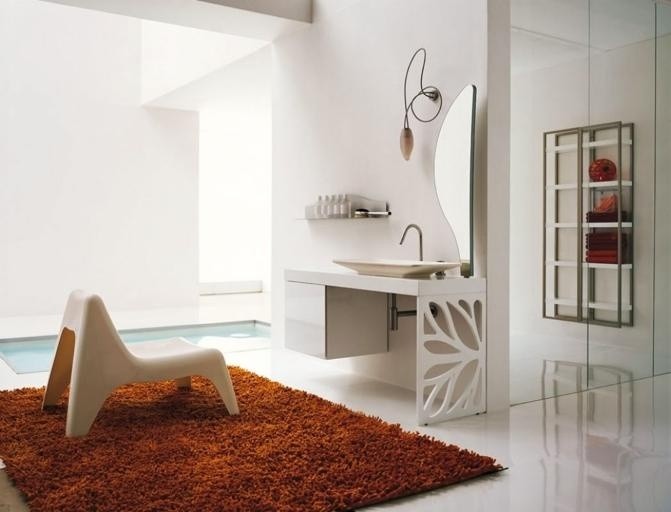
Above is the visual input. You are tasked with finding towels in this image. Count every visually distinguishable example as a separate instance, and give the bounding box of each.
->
[585,210,627,264]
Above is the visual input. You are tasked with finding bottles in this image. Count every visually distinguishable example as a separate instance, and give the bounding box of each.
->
[313,193,351,219]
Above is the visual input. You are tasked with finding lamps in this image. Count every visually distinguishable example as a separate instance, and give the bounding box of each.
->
[399,48,442,161]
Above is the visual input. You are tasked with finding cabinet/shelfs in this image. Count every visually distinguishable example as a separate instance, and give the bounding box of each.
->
[283,268,487,427]
[542,360,634,512]
[542,122,634,328]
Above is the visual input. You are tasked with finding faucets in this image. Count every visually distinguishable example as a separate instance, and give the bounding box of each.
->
[400,222,425,260]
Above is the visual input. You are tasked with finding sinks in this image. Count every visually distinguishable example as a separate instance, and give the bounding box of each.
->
[333,255,459,277]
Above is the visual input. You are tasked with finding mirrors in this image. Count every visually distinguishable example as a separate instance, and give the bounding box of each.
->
[433,82,476,276]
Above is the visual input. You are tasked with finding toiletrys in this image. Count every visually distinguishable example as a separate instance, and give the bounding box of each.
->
[314,194,351,218]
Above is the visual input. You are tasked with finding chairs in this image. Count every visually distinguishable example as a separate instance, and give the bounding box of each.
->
[41,289,241,438]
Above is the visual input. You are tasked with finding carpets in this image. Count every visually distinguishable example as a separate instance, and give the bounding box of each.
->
[0,365,508,512]
